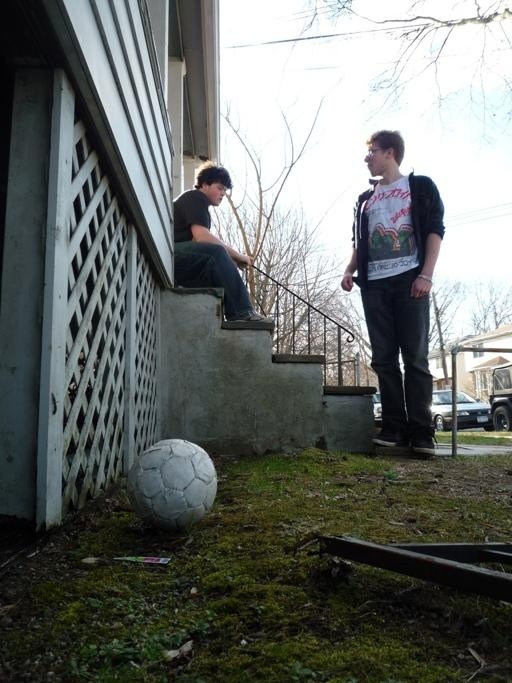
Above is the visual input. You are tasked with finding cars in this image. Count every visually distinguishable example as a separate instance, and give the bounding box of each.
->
[372,391,382,425]
[429,389,492,432]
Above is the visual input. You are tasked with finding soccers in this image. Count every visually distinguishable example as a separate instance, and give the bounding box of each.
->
[126,439,218,528]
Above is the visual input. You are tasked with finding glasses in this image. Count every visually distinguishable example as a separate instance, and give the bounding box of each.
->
[367,147,381,156]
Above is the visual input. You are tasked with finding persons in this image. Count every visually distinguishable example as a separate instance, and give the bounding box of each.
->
[175,159,275,324]
[341,130,446,454]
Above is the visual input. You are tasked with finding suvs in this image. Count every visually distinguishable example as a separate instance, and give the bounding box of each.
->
[488,360,512,431]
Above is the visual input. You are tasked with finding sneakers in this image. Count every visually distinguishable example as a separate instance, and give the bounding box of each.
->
[227,308,275,323]
[373,429,408,446]
[410,434,435,454]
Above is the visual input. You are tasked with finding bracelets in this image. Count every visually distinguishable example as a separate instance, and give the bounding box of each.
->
[418,274,433,284]
